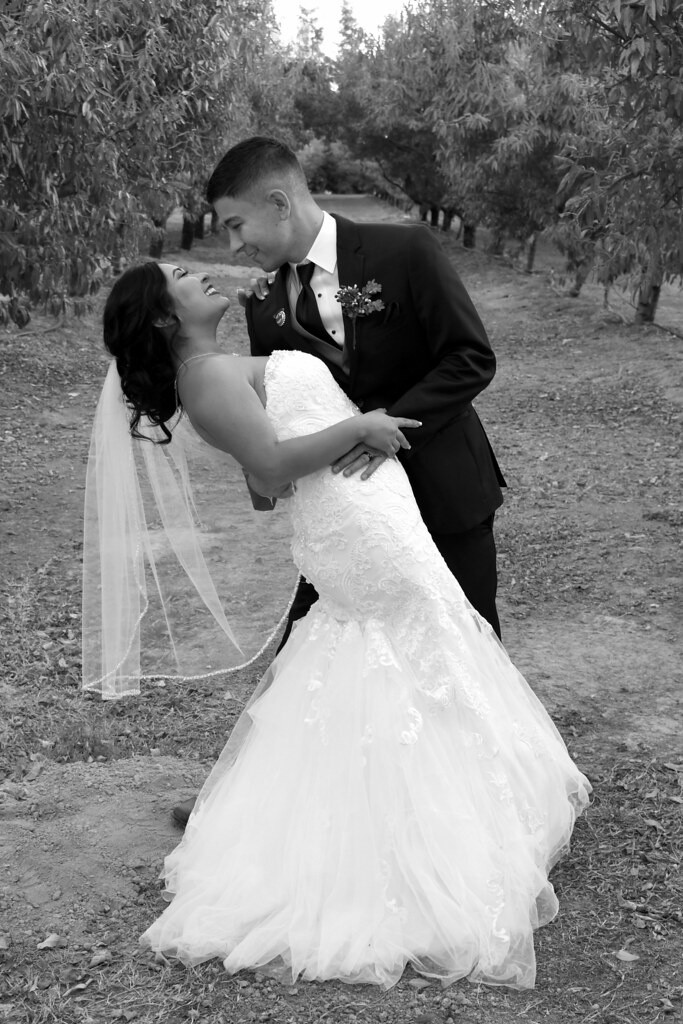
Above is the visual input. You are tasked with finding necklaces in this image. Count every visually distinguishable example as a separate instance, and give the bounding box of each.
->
[175,352,241,412]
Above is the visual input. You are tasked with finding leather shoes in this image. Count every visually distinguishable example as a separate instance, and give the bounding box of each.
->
[173,797,197,824]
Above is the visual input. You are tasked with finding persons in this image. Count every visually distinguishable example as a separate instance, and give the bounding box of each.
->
[170,132,512,824]
[104,259,596,995]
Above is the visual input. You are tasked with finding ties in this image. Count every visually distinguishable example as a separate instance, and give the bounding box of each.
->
[295,262,343,351]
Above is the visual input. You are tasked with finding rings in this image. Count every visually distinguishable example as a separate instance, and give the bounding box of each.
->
[363,451,372,461]
